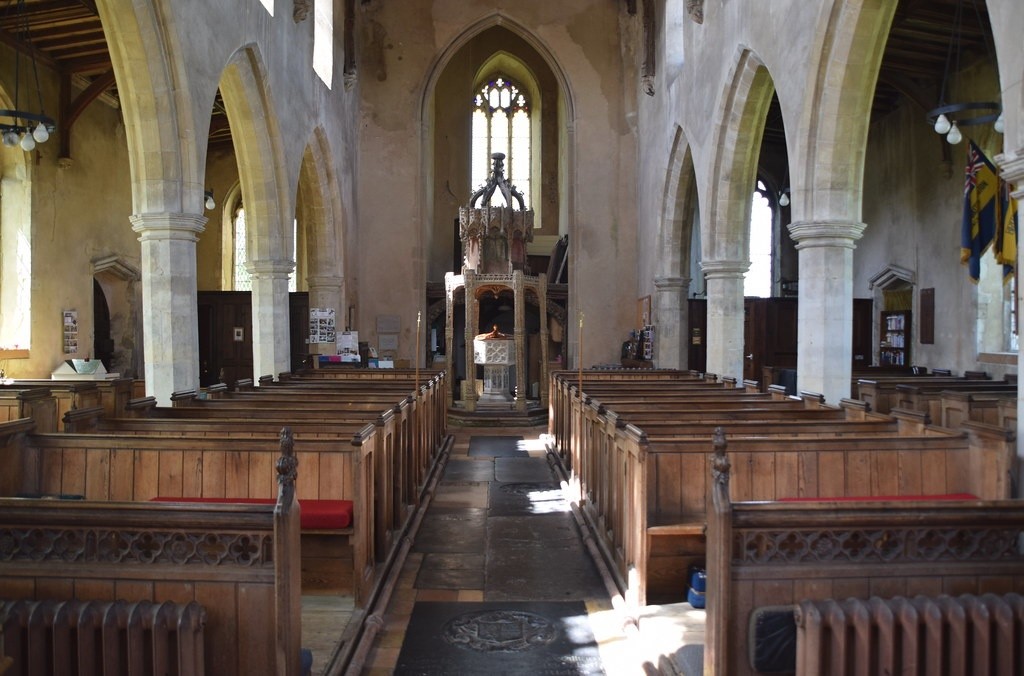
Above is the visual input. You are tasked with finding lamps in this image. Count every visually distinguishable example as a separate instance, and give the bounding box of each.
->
[927,0,1005,145]
[0,0,55,151]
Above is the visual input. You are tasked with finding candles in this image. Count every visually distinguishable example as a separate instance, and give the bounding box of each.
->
[580,319,583,327]
[417,310,422,325]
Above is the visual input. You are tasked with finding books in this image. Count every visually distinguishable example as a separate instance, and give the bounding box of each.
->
[880,333,905,348]
[887,315,904,330]
[880,350,905,365]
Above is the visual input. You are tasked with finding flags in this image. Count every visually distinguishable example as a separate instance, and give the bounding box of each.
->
[959,142,1019,285]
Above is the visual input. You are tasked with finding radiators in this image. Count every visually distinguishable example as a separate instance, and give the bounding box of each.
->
[0,596,210,676]
[792,593,1024,675]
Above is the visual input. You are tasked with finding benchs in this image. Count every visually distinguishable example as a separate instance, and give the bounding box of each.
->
[548,367,1018,601]
[0,362,448,605]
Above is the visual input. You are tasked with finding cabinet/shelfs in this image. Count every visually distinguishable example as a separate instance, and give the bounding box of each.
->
[880,308,912,366]
